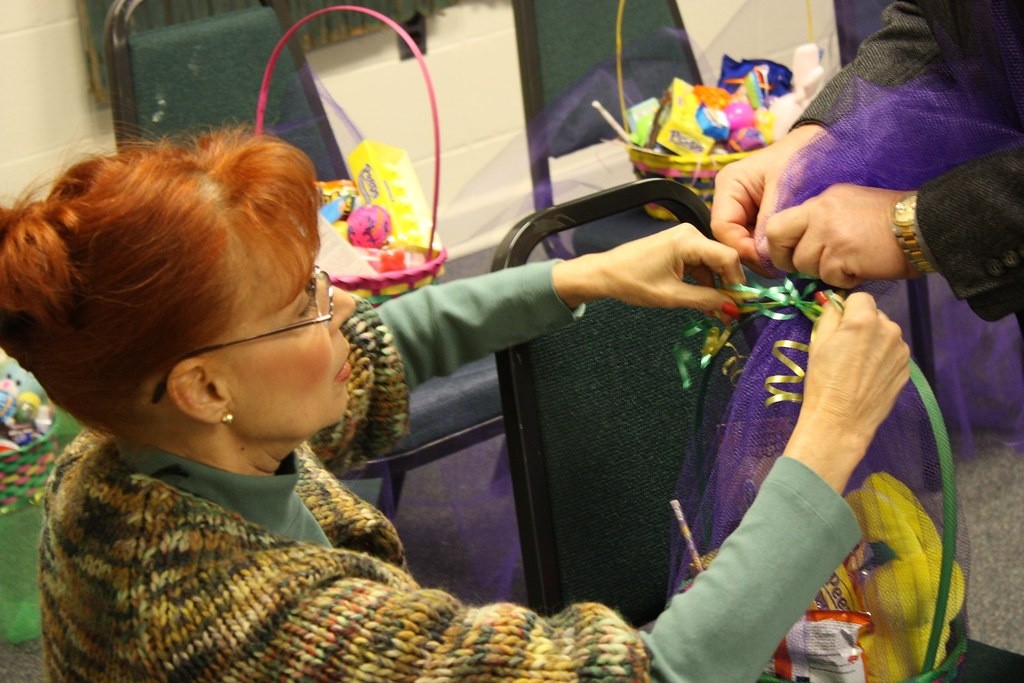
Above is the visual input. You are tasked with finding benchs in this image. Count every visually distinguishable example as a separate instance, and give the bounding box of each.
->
[333,220,577,521]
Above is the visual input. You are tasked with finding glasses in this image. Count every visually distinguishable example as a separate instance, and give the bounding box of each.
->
[150,265,333,405]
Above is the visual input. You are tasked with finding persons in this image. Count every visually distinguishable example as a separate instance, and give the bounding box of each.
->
[710,0,1024,322]
[1,128,911,683]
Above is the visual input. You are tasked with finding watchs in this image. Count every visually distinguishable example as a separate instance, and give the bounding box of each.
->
[891,192,938,274]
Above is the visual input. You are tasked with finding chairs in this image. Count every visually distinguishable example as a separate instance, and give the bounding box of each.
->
[512,0,944,492]
[492,177,760,627]
[103,0,510,520]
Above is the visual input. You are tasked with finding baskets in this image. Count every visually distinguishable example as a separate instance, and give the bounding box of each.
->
[254,7,447,309]
[616,0,814,224]
[693,302,968,683]
